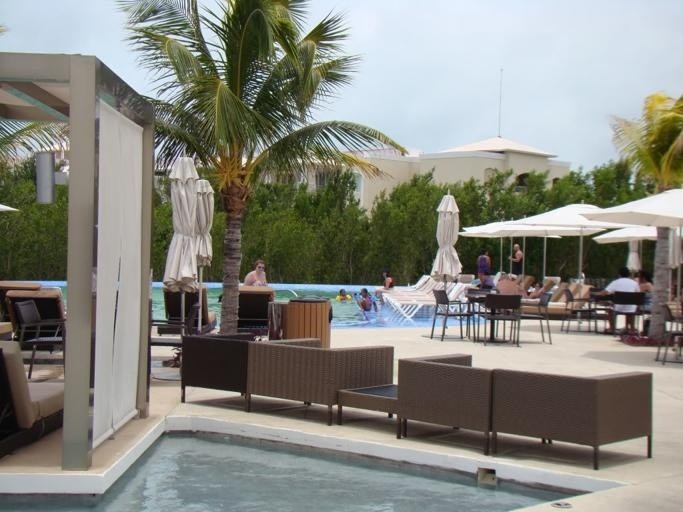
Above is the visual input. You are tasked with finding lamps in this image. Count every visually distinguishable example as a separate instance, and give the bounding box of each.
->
[33,148,67,207]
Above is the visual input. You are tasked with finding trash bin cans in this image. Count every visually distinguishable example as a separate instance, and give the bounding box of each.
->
[287,296,333,349]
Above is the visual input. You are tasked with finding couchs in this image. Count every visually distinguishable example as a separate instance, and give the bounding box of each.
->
[494,368,653,471]
[246,338,394,425]
[179,332,251,412]
[397,354,492,455]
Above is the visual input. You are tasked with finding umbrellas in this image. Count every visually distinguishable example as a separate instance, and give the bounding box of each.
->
[0,204,19,213]
[164,157,200,337]
[430,188,464,297]
[195,178,213,332]
[458,188,683,332]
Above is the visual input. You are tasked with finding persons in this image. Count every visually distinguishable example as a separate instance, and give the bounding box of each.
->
[243,260,267,286]
[529,280,545,298]
[600,268,654,334]
[507,244,523,278]
[476,248,492,288]
[336,271,393,312]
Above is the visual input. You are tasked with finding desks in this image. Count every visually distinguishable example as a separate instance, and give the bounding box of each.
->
[465,293,508,344]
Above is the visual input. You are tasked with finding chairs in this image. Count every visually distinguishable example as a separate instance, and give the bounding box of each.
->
[160,284,218,334]
[656,302,682,363]
[477,294,522,347]
[0,280,66,459]
[430,289,464,341]
[606,291,647,337]
[237,286,275,331]
[381,272,596,333]
[511,291,553,346]
